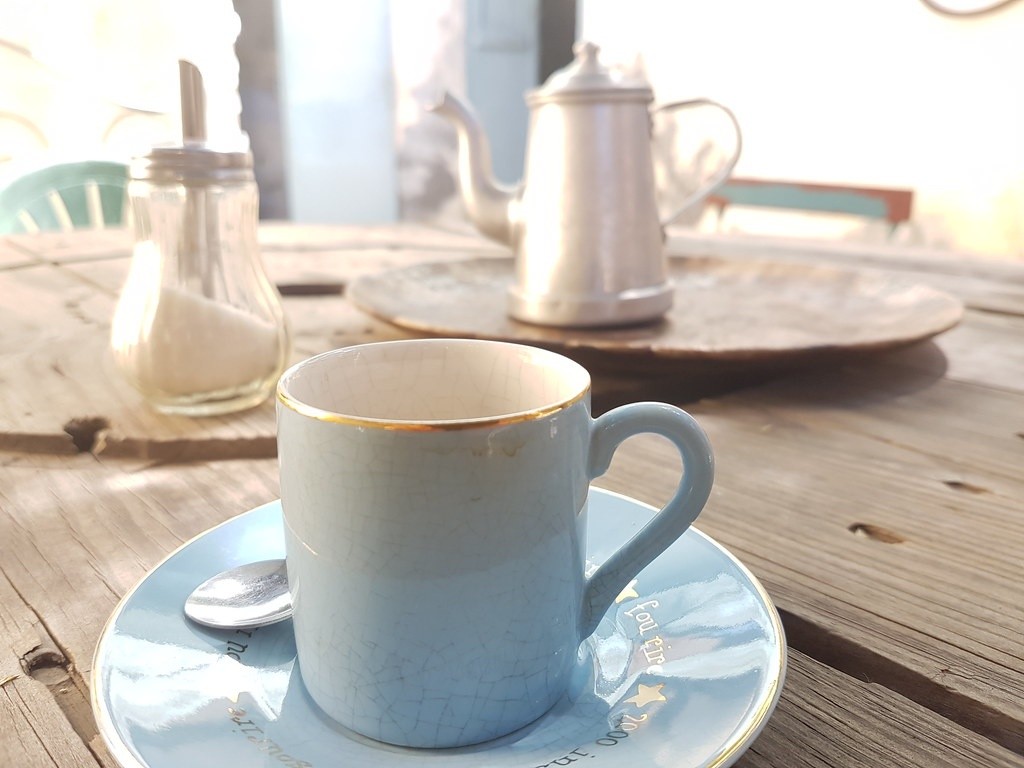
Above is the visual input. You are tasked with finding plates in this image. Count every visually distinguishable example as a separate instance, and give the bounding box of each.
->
[88,480,786,767]
[343,244,967,363]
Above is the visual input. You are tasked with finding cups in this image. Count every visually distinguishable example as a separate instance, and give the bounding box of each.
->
[275,338,715,748]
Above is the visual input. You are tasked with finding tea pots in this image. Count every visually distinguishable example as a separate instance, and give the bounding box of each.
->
[428,40,746,327]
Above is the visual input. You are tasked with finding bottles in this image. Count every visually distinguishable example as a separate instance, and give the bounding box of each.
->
[116,148,284,419]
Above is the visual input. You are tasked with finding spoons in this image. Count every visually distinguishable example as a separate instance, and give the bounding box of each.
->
[184,559,296,629]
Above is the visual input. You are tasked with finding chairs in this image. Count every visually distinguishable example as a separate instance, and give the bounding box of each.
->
[709,178,916,229]
[0,161,128,235]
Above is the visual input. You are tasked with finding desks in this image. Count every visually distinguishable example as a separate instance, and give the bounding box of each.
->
[0,226,1024,768]
